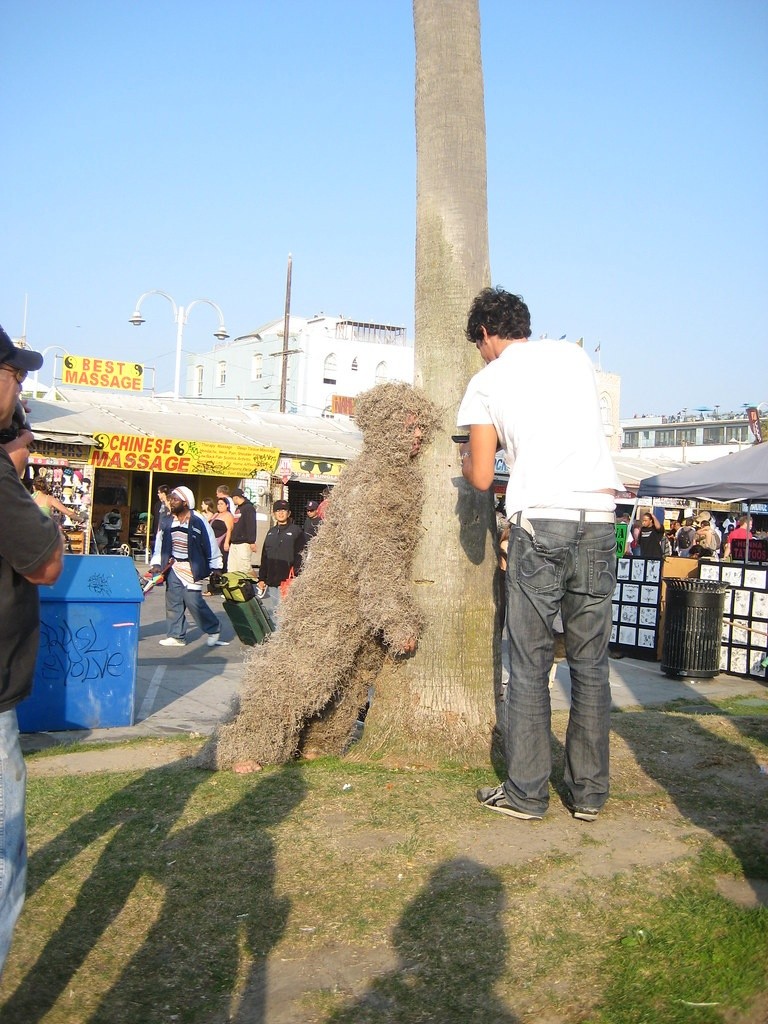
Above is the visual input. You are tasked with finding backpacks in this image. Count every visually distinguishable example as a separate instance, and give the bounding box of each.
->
[678,528,692,549]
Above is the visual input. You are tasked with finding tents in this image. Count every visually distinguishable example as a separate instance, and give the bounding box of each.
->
[626,439,768,563]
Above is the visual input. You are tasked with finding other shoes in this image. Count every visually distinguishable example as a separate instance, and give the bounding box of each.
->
[202,592,212,596]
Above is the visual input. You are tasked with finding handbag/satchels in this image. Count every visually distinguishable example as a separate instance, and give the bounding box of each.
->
[279,566,296,600]
[221,571,257,603]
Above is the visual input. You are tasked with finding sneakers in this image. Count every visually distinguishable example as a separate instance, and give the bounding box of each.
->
[567,794,599,821]
[158,638,185,646]
[207,621,221,646]
[256,582,268,598]
[476,782,544,821]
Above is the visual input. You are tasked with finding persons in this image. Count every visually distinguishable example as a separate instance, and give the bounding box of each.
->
[149,484,269,599]
[621,511,754,560]
[63,469,90,526]
[191,383,439,775]
[259,500,323,609]
[459,285,627,823]
[30,476,84,523]
[634,409,768,424]
[149,485,224,647]
[0,321,64,978]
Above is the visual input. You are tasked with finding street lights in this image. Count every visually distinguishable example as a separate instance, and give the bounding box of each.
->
[128,290,230,400]
[728,428,751,453]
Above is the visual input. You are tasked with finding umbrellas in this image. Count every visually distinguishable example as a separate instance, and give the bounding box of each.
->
[693,405,713,413]
[738,402,757,408]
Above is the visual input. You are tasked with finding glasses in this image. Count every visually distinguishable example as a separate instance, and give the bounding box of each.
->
[306,510,314,512]
[0,367,28,382]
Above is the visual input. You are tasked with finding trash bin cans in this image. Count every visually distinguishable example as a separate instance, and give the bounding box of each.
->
[661,577,731,683]
[18,554,144,733]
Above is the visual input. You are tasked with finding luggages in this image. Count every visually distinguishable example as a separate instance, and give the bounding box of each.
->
[214,577,276,647]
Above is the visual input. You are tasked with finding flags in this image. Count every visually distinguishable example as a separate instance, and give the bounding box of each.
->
[746,408,762,444]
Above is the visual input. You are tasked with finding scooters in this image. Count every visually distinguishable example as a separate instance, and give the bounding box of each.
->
[97,520,132,557]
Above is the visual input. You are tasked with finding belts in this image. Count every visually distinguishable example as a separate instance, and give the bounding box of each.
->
[509,509,615,536]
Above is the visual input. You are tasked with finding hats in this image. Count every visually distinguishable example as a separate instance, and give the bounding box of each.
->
[273,500,289,512]
[305,500,318,510]
[229,489,244,497]
[0,324,44,371]
[172,486,195,509]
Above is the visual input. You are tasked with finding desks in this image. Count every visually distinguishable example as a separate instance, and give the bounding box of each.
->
[130,532,154,561]
